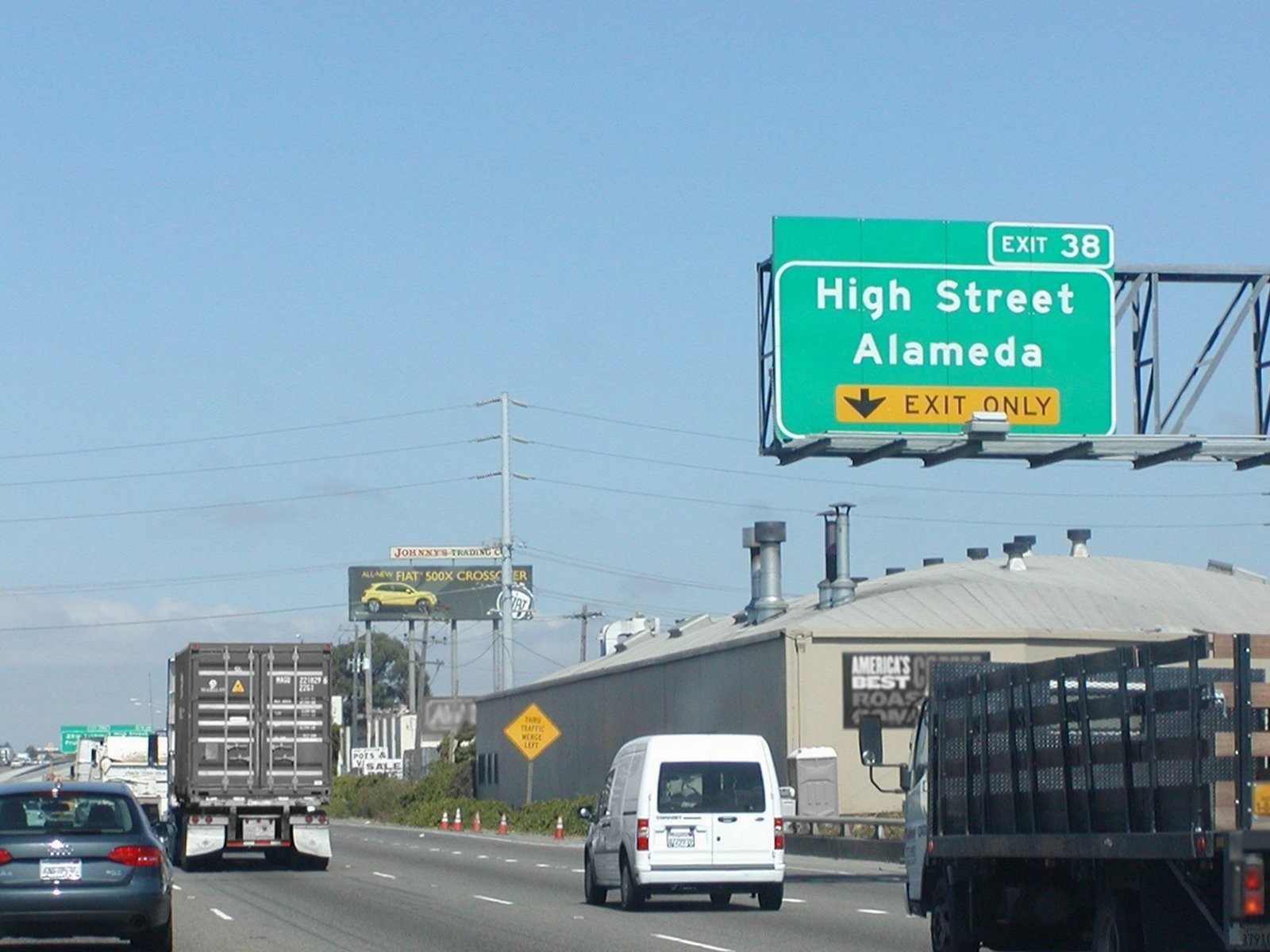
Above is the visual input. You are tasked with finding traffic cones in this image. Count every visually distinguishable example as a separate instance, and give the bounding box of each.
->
[498,812,508,836]
[554,816,565,839]
[440,811,449,831]
[452,808,463,831]
[470,810,481,832]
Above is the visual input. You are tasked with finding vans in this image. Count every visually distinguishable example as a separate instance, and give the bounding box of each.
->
[577,734,795,913]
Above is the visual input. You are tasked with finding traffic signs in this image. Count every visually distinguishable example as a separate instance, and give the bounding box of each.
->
[775,260,1118,441]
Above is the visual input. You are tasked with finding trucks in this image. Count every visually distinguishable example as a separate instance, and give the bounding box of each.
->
[856,631,1270,951]
[165,640,334,871]
[74,735,170,831]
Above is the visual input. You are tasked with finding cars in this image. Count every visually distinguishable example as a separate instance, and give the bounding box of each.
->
[0,781,176,952]
[8,756,33,769]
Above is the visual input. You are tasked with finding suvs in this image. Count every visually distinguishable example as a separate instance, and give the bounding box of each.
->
[360,581,438,614]
